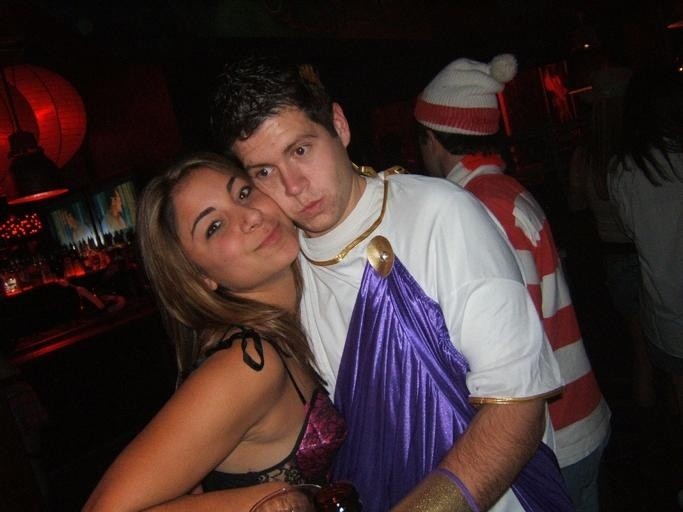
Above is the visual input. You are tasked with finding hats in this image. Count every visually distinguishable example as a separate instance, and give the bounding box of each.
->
[410,50,520,137]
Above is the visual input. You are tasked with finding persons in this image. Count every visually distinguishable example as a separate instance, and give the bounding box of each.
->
[101,187,127,236]
[64,204,92,240]
[606,64,683,378]
[80,156,349,511]
[566,91,683,414]
[206,50,577,512]
[409,53,614,512]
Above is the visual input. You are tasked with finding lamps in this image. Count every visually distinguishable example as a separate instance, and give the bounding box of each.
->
[1,69,76,206]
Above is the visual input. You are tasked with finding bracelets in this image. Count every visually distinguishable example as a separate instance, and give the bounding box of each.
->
[435,466,482,512]
